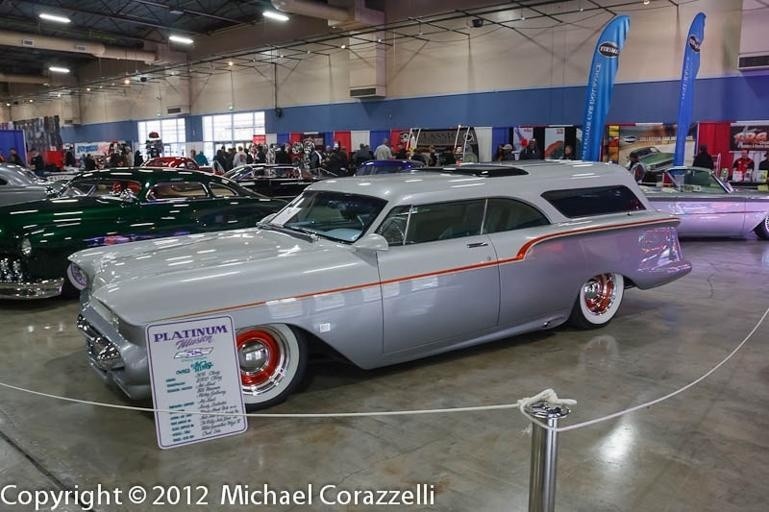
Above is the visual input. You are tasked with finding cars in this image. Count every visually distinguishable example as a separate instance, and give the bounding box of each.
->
[67,159,693,410]
[112,156,224,197]
[0,163,84,205]
[641,165,769,240]
[0,167,298,307]
[623,135,637,142]
[434,147,454,164]
[220,163,324,203]
[327,159,426,222]
[630,146,676,179]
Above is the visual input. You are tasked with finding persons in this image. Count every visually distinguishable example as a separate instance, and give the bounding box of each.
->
[758,149,769,169]
[9,148,21,165]
[732,149,754,174]
[520,138,540,160]
[65,146,143,169]
[564,146,575,158]
[214,139,441,178]
[29,148,44,176]
[627,151,645,184]
[692,144,714,168]
[499,145,514,160]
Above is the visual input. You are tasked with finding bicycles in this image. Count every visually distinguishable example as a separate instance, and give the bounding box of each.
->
[79,156,107,171]
